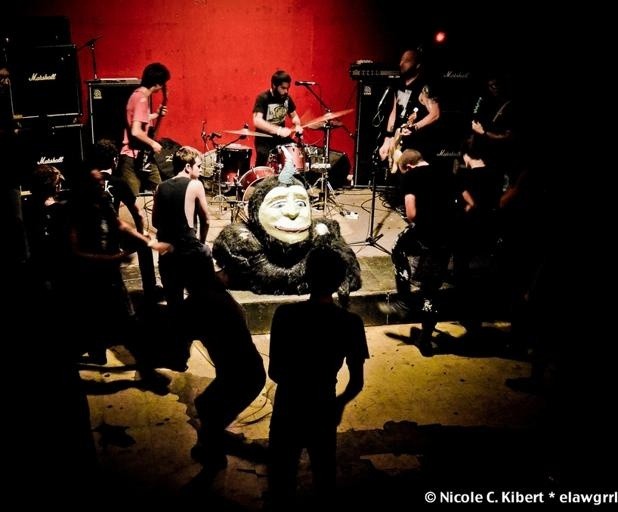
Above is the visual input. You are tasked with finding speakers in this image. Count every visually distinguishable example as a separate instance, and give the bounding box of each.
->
[14,189,99,259]
[14,123,85,196]
[87,79,143,148]
[2,44,81,121]
[353,80,399,188]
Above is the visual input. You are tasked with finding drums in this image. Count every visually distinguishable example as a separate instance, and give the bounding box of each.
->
[269,142,304,176]
[236,167,275,219]
[218,145,252,180]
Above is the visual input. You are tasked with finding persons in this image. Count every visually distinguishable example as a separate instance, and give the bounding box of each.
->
[152,147,210,345]
[67,158,130,364]
[252,70,302,167]
[469,75,532,208]
[94,139,143,236]
[158,251,270,497]
[392,149,455,320]
[118,64,169,196]
[268,244,369,512]
[366,47,440,187]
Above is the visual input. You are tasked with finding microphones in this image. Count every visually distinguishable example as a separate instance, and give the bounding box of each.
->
[295,81,320,86]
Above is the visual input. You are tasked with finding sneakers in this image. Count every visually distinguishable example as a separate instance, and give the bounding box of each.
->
[190,446,227,471]
[378,298,439,320]
[410,326,432,357]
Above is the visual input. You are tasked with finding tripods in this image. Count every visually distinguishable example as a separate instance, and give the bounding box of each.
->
[208,170,230,213]
[310,176,345,220]
[308,157,340,207]
[346,160,392,255]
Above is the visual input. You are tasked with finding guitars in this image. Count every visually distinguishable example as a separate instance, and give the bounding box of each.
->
[139,84,167,180]
[375,111,418,174]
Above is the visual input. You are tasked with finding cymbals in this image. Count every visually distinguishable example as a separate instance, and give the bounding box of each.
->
[308,121,345,130]
[301,109,353,128]
[221,129,273,137]
